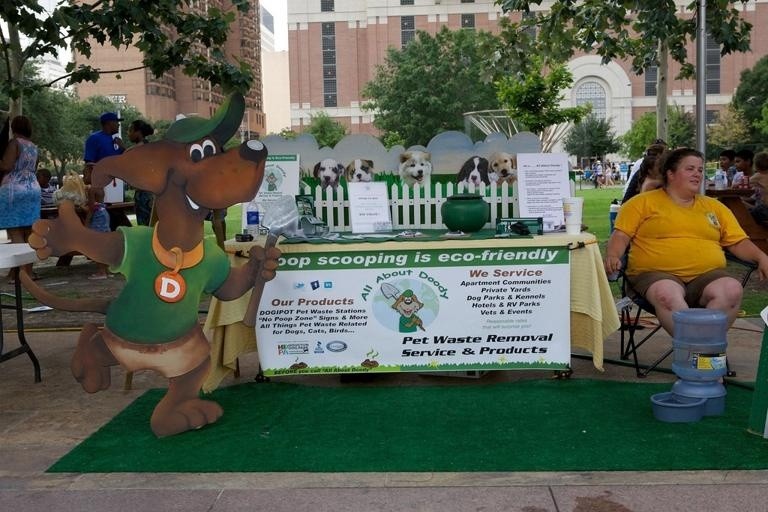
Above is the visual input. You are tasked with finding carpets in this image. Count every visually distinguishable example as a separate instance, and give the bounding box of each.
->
[44,385,768,475]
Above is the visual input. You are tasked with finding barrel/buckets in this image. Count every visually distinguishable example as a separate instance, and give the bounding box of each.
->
[672,309,731,386]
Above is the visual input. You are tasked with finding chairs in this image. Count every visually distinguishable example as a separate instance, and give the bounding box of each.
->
[606,241,759,378]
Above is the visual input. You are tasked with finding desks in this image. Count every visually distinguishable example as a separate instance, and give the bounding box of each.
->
[40,201,136,266]
[704,188,768,251]
[200,229,620,394]
[0,241,41,382]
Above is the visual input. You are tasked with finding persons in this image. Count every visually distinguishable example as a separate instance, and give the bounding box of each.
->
[595,145,768,340]
[73,162,116,282]
[575,139,768,228]
[0,114,43,284]
[114,119,160,226]
[34,168,59,205]
[83,111,126,168]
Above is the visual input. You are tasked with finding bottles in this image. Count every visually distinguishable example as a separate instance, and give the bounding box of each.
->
[245,198,260,239]
[713,170,725,189]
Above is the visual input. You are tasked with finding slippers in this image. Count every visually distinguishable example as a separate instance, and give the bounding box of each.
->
[88,274,107,280]
[107,273,114,278]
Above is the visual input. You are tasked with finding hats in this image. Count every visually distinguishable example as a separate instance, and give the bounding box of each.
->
[100,113,124,122]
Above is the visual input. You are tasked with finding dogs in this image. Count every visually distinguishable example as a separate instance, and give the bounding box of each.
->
[344,159,374,182]
[456,155,498,189]
[313,159,344,191]
[488,152,517,184]
[399,150,432,188]
